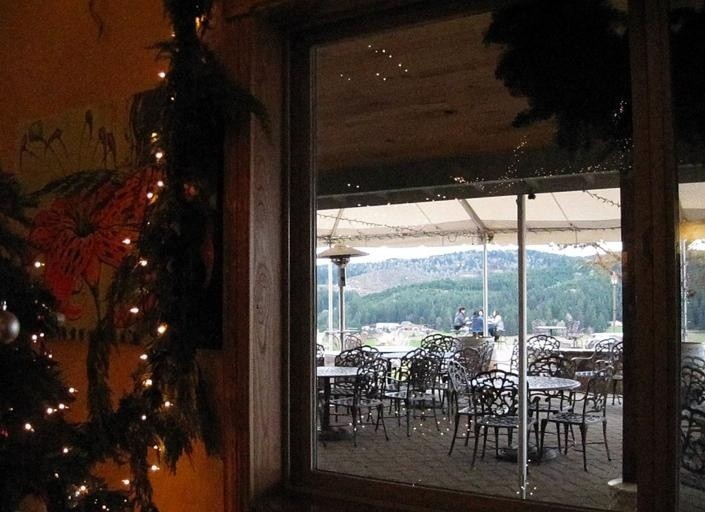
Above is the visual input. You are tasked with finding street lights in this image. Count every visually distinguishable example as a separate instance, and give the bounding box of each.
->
[610,271,618,327]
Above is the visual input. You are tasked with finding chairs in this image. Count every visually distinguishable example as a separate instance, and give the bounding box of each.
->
[316,316,705,491]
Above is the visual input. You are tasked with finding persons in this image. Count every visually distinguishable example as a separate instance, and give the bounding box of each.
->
[489,308,505,342]
[471,311,483,336]
[479,309,483,317]
[453,306,471,337]
